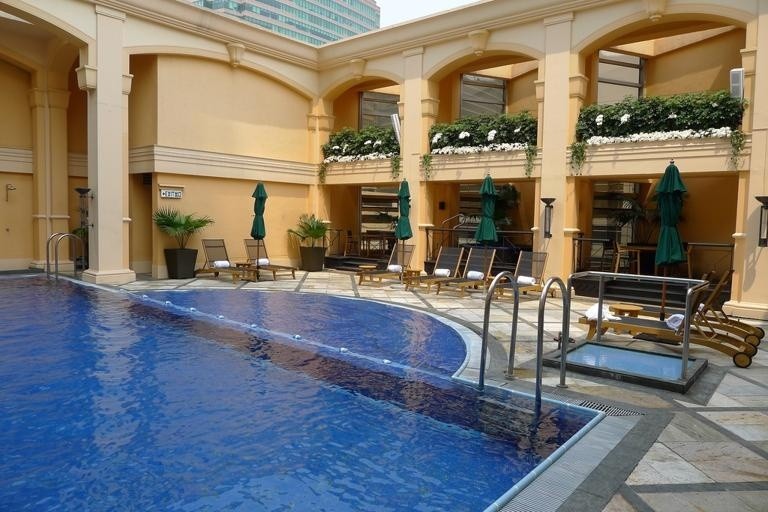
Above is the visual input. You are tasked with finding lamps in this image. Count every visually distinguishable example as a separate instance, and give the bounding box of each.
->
[540,196,556,238]
[754,194,768,247]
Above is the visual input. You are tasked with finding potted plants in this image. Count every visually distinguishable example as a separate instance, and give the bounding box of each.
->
[285,213,332,272]
[151,204,215,281]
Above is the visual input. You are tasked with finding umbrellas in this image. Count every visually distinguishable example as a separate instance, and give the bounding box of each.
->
[653,159,689,322]
[250,182,269,279]
[474,172,498,294]
[393,178,414,286]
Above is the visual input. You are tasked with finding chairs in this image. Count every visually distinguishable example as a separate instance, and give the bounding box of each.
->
[192,236,298,287]
[578,239,765,369]
[343,229,550,304]
